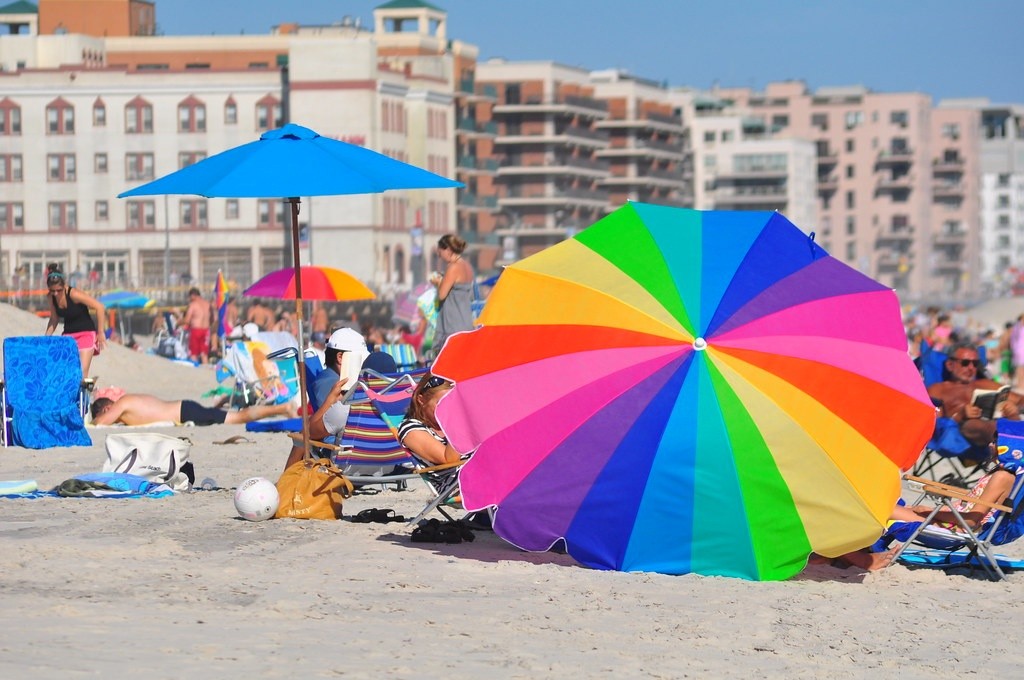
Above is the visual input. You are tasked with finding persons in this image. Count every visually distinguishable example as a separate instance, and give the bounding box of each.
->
[807,307,1024,571]
[45,235,488,509]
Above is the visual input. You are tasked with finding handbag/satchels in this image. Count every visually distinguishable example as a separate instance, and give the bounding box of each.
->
[417,285,441,328]
[274,458,352,521]
[103,432,195,493]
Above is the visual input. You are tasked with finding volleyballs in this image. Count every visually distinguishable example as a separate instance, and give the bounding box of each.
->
[234,476,280,521]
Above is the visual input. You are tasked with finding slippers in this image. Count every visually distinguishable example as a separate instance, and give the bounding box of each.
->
[212,435,257,444]
[411,525,435,541]
[358,508,404,523]
[436,524,462,544]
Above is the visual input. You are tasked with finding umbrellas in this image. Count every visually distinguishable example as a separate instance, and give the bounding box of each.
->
[96,290,157,349]
[212,269,233,361]
[431,199,937,584]
[388,282,437,329]
[116,124,467,459]
[242,266,377,344]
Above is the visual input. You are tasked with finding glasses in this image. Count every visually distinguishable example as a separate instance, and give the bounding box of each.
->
[419,375,445,396]
[49,287,64,293]
[949,357,981,367]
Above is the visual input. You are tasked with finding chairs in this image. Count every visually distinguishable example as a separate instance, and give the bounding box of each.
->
[214,332,497,533]
[885,336,1024,585]
[0,336,94,450]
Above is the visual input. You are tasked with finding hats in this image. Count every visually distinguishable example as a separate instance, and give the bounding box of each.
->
[360,351,397,375]
[242,323,258,338]
[326,328,368,352]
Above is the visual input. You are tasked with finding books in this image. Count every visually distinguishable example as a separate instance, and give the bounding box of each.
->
[969,384,1012,421]
